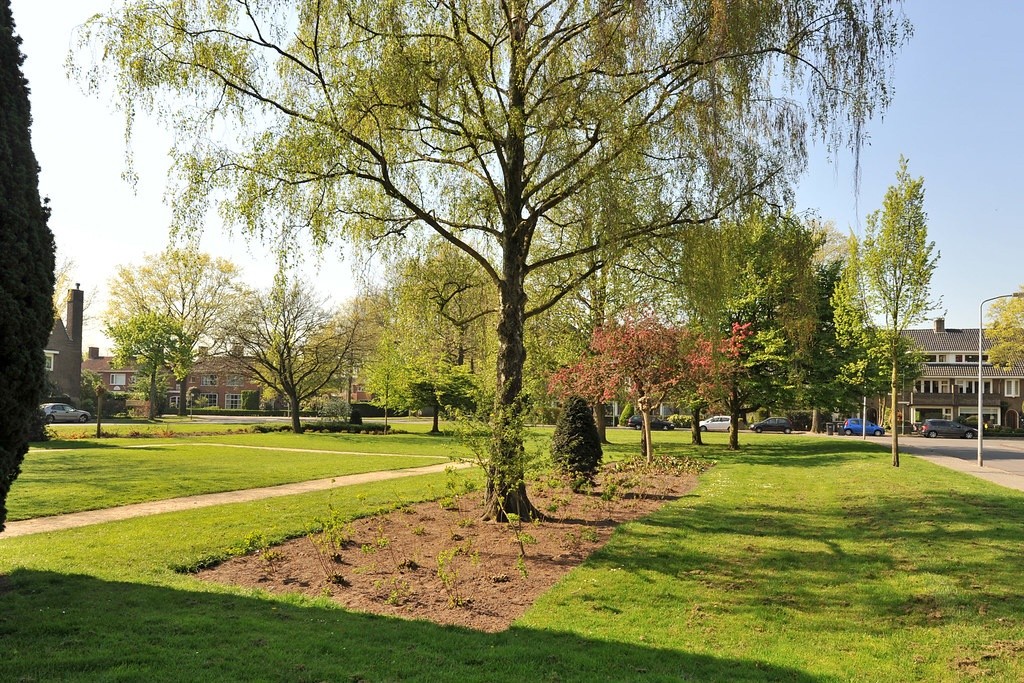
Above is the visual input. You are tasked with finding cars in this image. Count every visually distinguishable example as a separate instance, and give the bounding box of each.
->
[40,403,91,423]
[844,418,885,436]
[751,416,793,434]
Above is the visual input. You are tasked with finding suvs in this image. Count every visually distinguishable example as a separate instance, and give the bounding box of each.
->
[699,416,731,431]
[920,419,978,439]
[628,415,674,431]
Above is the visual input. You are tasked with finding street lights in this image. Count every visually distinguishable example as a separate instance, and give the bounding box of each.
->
[977,292,1024,467]
